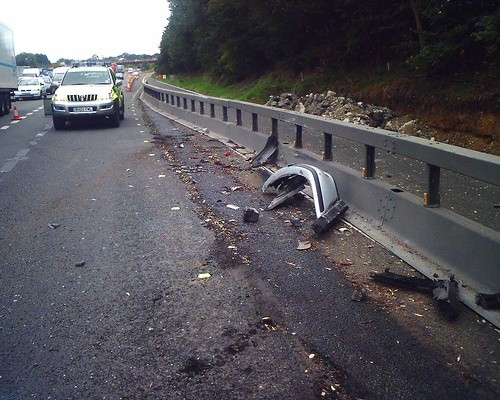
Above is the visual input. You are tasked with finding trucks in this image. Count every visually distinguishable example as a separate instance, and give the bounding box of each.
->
[0,23,19,116]
[49,66,73,95]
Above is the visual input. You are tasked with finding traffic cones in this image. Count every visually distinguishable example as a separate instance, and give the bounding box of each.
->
[11,105,22,120]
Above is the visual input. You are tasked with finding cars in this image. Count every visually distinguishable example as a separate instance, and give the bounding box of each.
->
[13,78,47,100]
[15,66,53,94]
[110,62,141,92]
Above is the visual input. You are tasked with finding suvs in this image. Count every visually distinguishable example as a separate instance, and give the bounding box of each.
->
[50,62,125,130]
